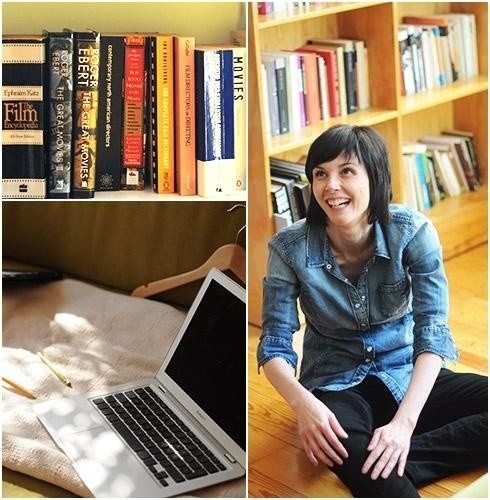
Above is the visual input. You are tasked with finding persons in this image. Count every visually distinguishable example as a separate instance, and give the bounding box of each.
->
[256,125,488,498]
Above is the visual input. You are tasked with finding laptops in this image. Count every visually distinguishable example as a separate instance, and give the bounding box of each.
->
[34,267,246,497]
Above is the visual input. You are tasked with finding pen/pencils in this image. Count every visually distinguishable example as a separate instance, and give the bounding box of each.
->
[39,356,71,387]
[2,377,37,399]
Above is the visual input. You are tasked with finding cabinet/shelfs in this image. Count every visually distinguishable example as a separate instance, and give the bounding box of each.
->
[248,2,487,327]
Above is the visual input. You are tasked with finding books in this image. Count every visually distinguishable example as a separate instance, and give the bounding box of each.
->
[255,3,481,236]
[4,31,247,201]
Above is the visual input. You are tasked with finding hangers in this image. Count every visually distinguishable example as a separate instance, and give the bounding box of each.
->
[131,204,246,297]
